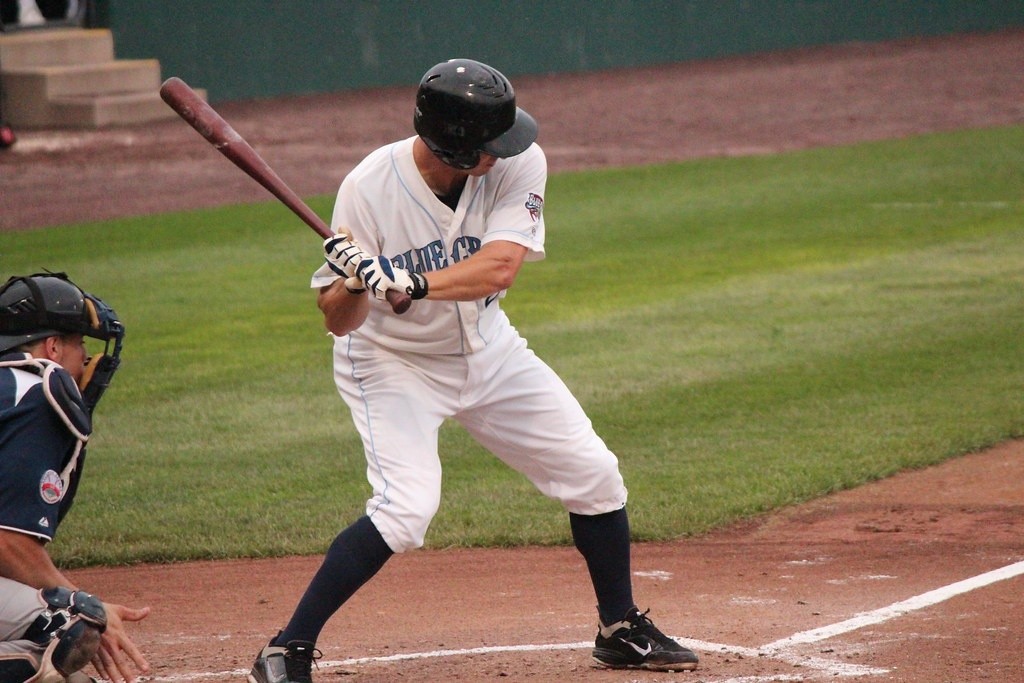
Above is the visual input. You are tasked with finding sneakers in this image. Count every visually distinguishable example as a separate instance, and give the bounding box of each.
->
[248,630,324,683]
[591,607,701,671]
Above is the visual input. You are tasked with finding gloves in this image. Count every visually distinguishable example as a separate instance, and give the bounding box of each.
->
[344,255,426,306]
[321,233,373,279]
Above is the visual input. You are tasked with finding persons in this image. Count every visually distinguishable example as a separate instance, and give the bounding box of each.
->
[0,266,151,683]
[246,59,699,683]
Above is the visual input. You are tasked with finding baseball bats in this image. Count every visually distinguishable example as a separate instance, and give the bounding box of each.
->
[155,72,417,318]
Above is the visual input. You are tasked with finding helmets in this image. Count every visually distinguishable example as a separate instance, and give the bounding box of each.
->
[0,270,124,357]
[411,57,539,172]
